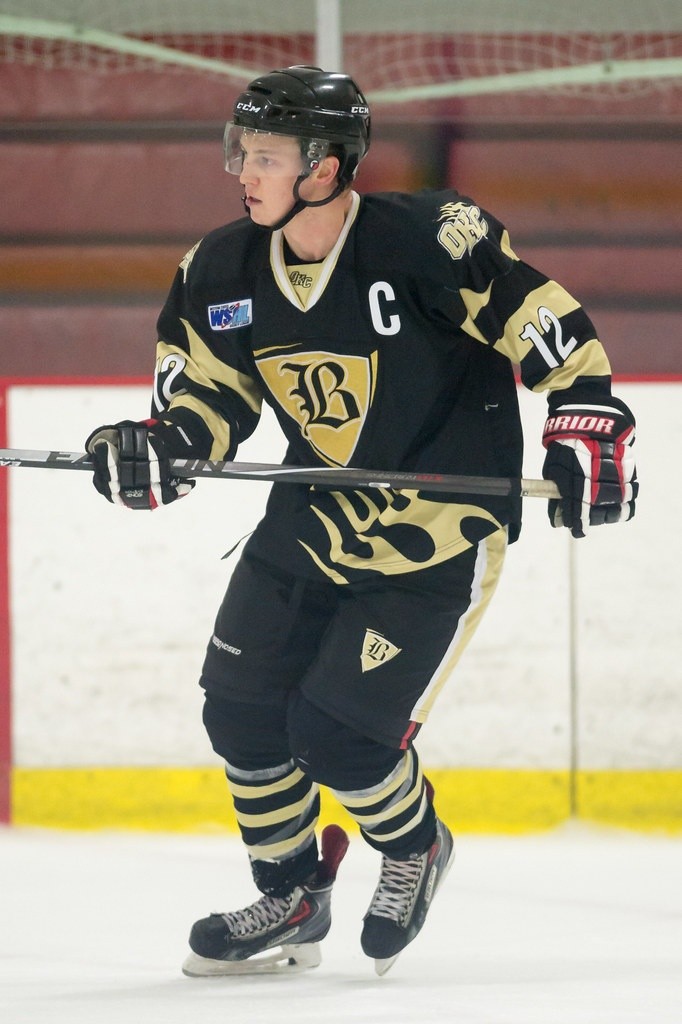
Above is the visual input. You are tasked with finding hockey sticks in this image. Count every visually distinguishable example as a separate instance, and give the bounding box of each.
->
[0,447,562,506]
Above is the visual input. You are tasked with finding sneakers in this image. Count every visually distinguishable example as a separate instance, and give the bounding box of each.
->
[182,825,349,976]
[360,774,456,976]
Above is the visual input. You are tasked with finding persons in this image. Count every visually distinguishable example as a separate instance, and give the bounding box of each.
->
[84,63,641,978]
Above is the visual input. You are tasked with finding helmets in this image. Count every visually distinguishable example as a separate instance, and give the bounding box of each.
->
[222,65,373,183]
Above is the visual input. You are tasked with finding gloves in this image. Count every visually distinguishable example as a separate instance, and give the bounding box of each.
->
[542,397,639,538]
[86,413,202,511]
